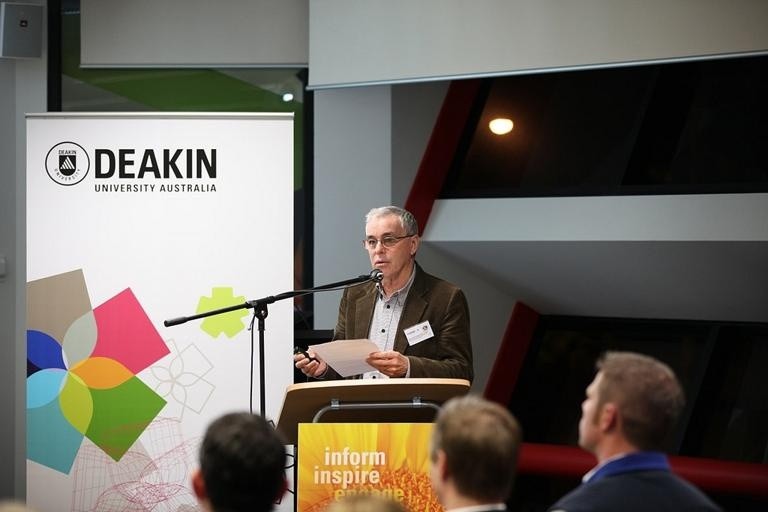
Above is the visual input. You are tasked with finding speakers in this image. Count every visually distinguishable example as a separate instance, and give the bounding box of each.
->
[0,1,44,60]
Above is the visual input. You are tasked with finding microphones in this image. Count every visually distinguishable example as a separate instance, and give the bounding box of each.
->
[370,268,384,281]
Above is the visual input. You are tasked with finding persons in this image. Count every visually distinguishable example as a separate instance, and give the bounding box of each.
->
[292,205,477,388]
[424,393,522,512]
[542,350,728,512]
[191,411,291,512]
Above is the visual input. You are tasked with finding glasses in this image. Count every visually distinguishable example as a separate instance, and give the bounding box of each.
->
[361,235,413,250]
